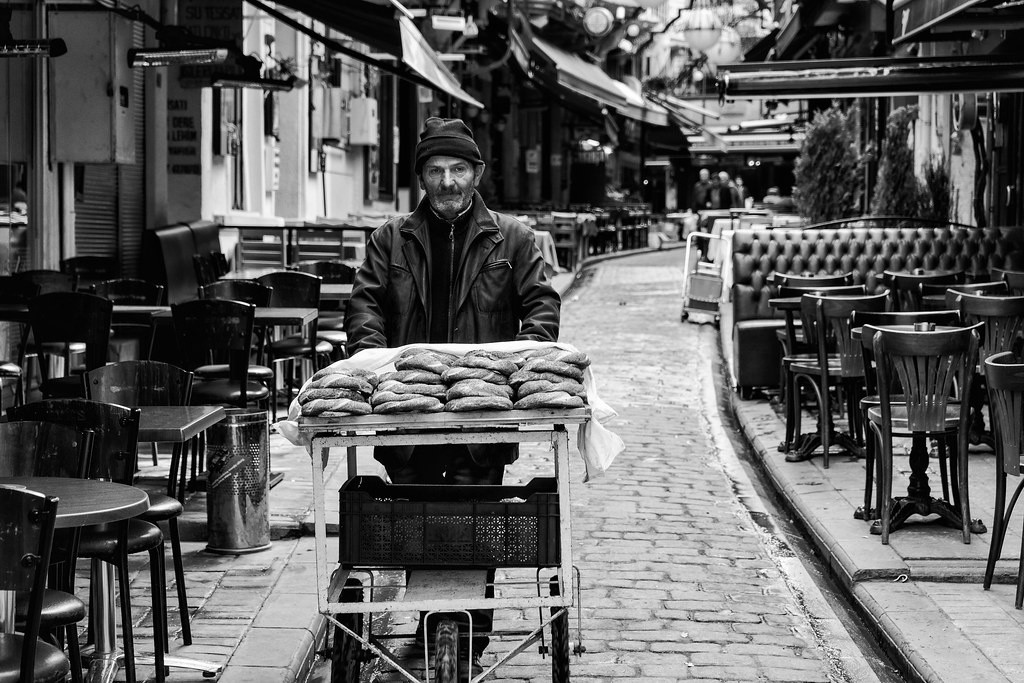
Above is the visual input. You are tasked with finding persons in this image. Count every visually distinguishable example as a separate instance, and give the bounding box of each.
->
[343,117,562,683]
[692,168,750,264]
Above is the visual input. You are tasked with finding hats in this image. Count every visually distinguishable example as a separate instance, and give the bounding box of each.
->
[414,117,484,175]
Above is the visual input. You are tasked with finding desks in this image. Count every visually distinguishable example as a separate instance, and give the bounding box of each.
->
[270,282,353,409]
[112,305,319,493]
[0,477,149,683]
[875,272,958,313]
[765,274,846,418]
[768,296,869,463]
[851,324,988,536]
[65,406,226,683]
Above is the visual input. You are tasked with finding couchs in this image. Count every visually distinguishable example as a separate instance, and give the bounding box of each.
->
[726,227,1024,400]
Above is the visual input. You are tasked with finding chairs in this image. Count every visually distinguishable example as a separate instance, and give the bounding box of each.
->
[0,200,655,683]
[984,351,1024,610]
[802,289,891,469]
[945,288,1024,451]
[849,310,964,521]
[779,284,867,455]
[991,268,1024,351]
[861,320,987,544]
[884,269,966,397]
[775,272,854,453]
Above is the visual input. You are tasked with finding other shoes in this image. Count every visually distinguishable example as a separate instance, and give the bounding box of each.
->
[416,623,435,647]
[456,652,485,683]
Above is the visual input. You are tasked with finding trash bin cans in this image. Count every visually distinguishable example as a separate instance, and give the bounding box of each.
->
[204,408,273,555]
[550,220,576,273]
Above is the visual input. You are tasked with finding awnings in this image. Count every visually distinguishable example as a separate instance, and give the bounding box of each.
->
[246,0,485,109]
[715,54,1024,97]
[521,32,628,112]
[606,77,669,127]
[891,0,1024,46]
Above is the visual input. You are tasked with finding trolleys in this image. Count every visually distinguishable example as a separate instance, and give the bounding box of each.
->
[299,407,586,683]
